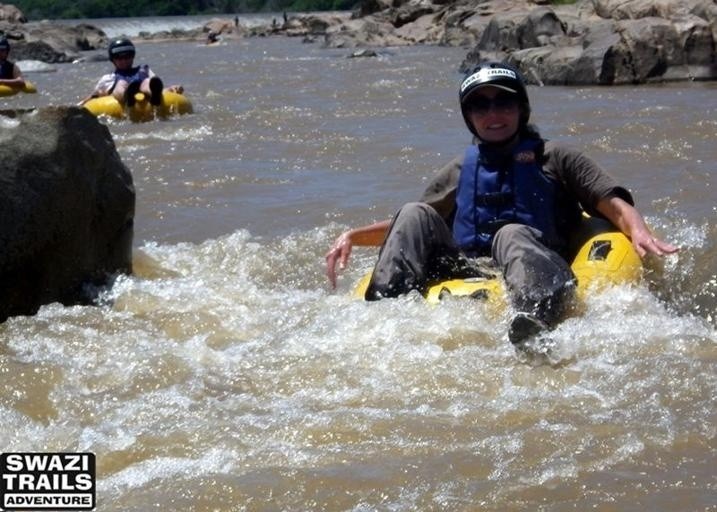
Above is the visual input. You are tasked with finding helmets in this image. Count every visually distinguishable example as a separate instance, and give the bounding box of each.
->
[0,39,11,51]
[108,38,135,57]
[458,62,523,106]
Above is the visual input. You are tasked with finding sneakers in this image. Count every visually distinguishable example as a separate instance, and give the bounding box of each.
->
[507,312,545,344]
[125,77,162,107]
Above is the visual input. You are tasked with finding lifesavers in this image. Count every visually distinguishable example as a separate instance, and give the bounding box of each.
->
[0,80,36,97]
[83,90,193,121]
[352,231,645,325]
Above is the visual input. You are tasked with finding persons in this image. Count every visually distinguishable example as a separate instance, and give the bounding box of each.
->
[0,35,27,88]
[322,62,679,363]
[83,36,184,112]
